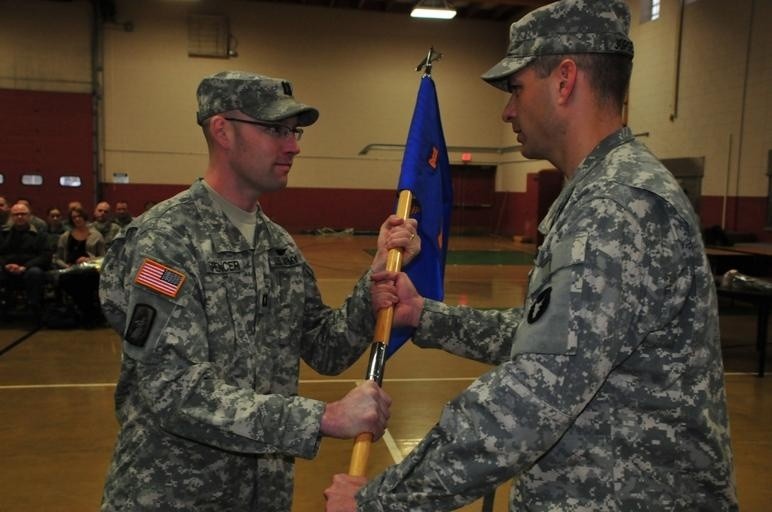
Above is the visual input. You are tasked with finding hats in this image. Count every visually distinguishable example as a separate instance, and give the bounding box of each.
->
[193,67,320,128]
[478,2,637,94]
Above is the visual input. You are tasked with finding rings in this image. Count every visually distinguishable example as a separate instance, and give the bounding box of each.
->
[410,233,415,239]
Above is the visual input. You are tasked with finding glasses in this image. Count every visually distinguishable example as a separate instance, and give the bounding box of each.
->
[221,115,305,142]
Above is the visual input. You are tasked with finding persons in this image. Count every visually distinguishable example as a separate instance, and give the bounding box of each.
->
[320,0,742,511]
[0,196,161,331]
[97,68,422,511]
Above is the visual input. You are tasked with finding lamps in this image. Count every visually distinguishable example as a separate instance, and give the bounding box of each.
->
[410,0,458,20]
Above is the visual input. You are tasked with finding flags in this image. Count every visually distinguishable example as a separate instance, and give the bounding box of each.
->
[385,74,456,366]
